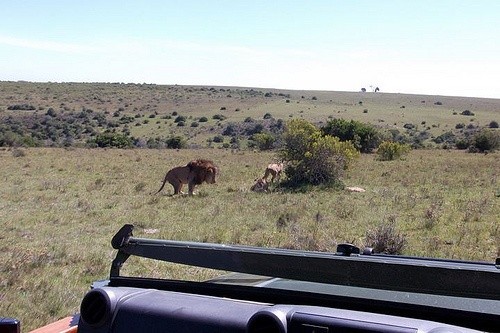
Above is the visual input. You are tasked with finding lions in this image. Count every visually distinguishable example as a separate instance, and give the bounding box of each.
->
[155,160,218,195]
